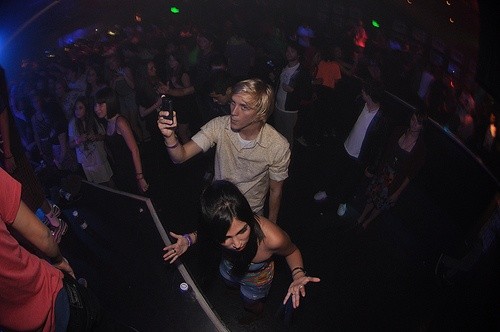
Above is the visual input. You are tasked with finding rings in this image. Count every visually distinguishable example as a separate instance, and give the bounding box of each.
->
[172,249,176,253]
[300,286,303,288]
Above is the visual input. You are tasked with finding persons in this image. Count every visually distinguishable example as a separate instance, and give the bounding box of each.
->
[0,168,106,332]
[162,179,321,324]
[7,0,500,236]
[0,67,16,175]
[156,78,291,290]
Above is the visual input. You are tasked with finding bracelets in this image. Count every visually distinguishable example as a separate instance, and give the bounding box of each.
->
[290,267,305,278]
[3,155,12,159]
[184,233,193,246]
[136,172,143,176]
[168,143,178,148]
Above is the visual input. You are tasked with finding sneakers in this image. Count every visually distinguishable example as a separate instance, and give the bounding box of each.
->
[313,191,327,201]
[336,203,347,216]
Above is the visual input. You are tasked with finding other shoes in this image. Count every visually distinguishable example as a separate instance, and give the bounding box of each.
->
[350,221,368,237]
[296,134,308,147]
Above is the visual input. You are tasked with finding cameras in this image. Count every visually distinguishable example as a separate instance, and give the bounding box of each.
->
[161,97,174,125]
